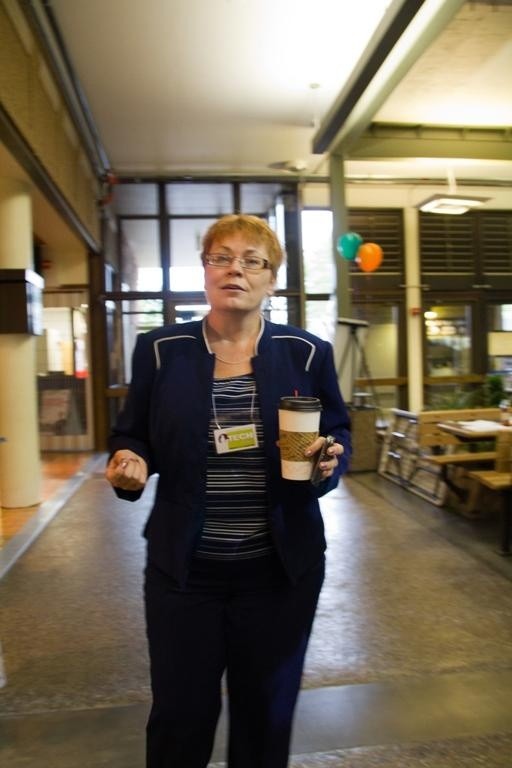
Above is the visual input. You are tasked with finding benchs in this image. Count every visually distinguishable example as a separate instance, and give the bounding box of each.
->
[419,407,511,521]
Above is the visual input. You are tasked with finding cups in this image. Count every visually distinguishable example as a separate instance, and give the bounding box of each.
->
[277,396,323,481]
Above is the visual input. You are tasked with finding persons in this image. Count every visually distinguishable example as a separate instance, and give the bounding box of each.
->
[103,210,354,766]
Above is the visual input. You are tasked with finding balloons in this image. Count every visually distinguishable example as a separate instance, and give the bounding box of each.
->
[355,242,386,277]
[337,231,365,262]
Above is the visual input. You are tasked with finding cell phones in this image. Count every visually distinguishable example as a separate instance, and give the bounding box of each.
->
[309,434,337,487]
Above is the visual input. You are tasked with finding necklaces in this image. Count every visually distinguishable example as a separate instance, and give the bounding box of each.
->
[208,350,254,366]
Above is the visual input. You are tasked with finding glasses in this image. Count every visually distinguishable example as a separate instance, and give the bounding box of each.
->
[201,253,273,270]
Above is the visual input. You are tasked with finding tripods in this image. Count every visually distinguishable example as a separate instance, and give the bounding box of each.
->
[338,329,387,434]
